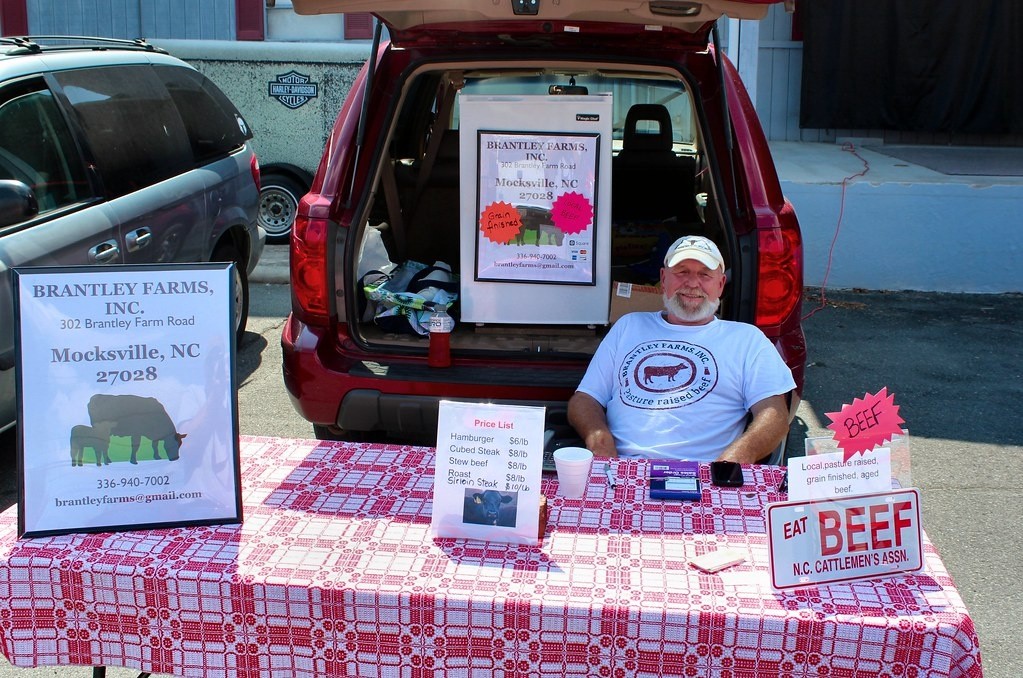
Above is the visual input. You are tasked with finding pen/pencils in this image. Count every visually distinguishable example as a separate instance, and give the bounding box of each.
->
[604,463,616,490]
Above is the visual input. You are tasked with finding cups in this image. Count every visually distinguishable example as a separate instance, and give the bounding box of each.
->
[552,448,593,500]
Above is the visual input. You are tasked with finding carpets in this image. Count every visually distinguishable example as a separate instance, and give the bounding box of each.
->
[866,145,1023,178]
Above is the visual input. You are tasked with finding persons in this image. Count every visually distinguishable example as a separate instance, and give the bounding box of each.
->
[567,235,798,463]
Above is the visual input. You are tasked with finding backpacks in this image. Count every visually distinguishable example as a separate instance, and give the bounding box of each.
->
[360,260,461,335]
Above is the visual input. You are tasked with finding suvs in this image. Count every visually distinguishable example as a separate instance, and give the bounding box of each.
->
[292,0,806,448]
[0,35,381,435]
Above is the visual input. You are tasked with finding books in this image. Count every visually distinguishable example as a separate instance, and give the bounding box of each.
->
[650,461,701,500]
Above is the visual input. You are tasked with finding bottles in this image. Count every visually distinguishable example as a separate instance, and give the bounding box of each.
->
[430,304,454,368]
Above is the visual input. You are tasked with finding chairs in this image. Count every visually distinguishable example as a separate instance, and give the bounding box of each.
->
[612,103,705,267]
[397,123,459,260]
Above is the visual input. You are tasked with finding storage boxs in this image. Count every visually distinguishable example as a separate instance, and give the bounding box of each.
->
[610,280,663,326]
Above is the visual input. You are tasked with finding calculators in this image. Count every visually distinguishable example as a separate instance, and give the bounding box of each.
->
[543,452,558,471]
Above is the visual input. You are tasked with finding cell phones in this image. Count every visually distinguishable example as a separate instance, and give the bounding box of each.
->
[711,460,744,487]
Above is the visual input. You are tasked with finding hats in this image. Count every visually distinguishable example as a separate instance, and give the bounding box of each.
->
[664,235,725,274]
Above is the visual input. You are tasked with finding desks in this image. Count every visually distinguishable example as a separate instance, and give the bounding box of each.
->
[0,436,985,678]
